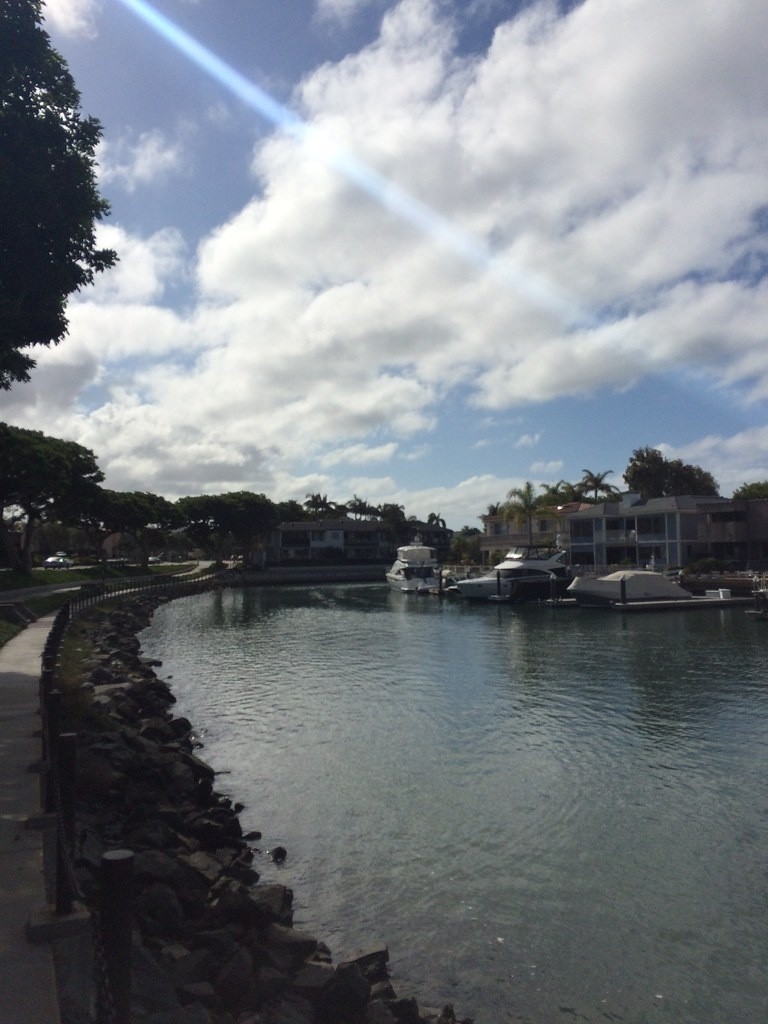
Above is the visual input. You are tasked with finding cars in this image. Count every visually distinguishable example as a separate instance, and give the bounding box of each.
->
[147,557,161,565]
[44,557,70,570]
[229,552,244,561]
[55,551,66,556]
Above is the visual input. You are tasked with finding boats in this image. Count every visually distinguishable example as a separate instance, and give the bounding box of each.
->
[385,544,447,594]
[455,559,573,603]
[565,570,692,609]
